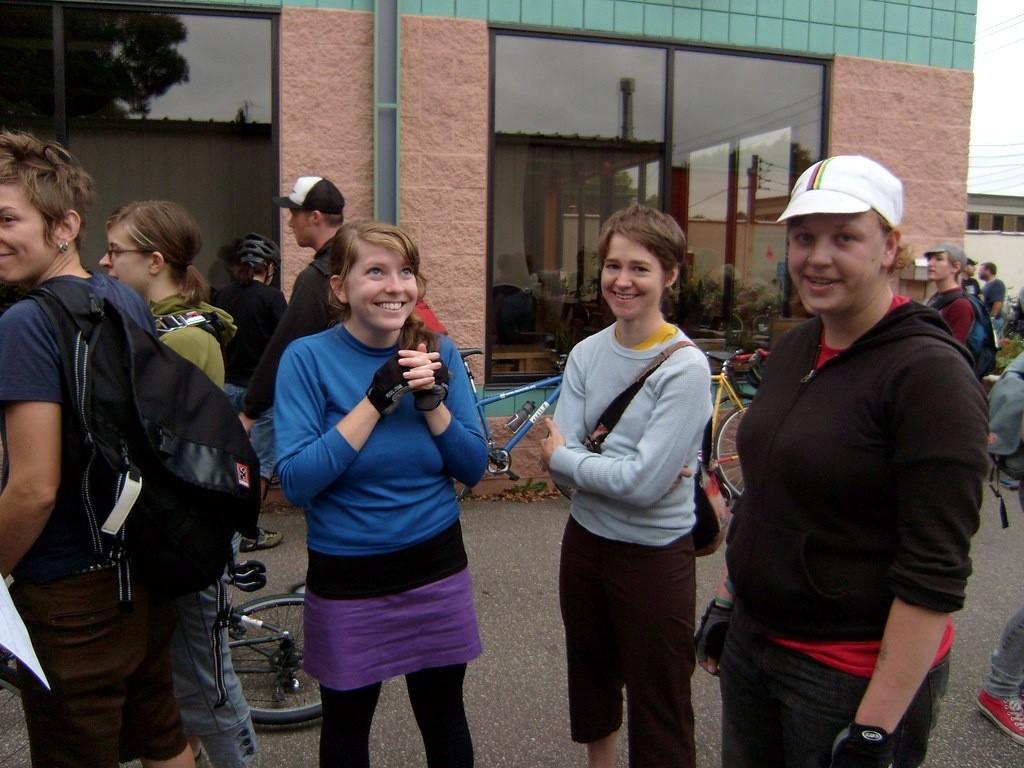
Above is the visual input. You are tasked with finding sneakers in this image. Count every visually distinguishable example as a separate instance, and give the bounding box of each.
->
[974,688,1020,743]
[238,531,282,552]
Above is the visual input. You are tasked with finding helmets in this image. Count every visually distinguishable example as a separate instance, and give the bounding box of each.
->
[238,233,281,267]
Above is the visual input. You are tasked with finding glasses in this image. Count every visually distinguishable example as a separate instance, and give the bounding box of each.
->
[107,243,169,264]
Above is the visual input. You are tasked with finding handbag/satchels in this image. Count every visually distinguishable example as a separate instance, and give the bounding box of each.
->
[692,452,726,556]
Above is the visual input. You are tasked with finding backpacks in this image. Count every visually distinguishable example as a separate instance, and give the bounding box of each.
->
[22,279,266,710]
[929,292,1002,382]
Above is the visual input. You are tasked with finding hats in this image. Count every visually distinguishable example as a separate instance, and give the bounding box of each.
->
[274,176,345,216]
[924,243,966,271]
[776,154,906,236]
[965,258,975,265]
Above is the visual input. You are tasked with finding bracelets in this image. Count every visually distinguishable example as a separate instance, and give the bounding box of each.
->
[714,596,734,606]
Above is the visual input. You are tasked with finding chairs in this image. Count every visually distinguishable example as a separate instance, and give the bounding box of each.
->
[492,285,538,344]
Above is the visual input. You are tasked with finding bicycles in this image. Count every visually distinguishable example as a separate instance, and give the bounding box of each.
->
[1,560,323,768]
[706,348,752,502]
[454,343,572,500]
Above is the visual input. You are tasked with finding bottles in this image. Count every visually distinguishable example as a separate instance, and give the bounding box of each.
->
[507,400,536,432]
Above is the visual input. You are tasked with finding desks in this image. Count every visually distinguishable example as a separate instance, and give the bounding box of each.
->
[493,343,561,373]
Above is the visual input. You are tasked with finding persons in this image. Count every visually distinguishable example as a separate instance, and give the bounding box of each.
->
[235,176,359,434]
[210,231,289,553]
[977,350,1024,745]
[695,153,985,768]
[0,128,201,767]
[271,217,490,768]
[533,204,717,768]
[920,240,1016,383]
[99,197,264,768]
